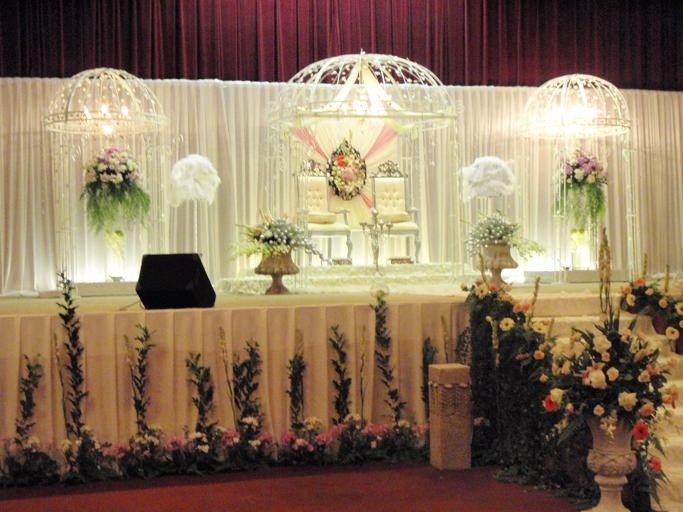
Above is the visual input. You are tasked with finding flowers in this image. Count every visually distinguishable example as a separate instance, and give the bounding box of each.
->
[459,211,546,264]
[229,207,335,267]
[1,267,682,512]
[325,138,369,199]
[79,151,151,236]
[550,151,608,233]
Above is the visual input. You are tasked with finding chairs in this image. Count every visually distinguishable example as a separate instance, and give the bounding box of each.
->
[369,159,422,268]
[290,171,356,267]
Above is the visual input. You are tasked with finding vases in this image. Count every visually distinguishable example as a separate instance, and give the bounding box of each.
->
[567,224,593,272]
[476,240,521,288]
[100,235,127,281]
[252,241,301,296]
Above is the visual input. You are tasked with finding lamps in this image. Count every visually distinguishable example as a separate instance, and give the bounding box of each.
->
[134,250,218,308]
[262,0,460,126]
[41,2,169,138]
[517,2,633,142]
